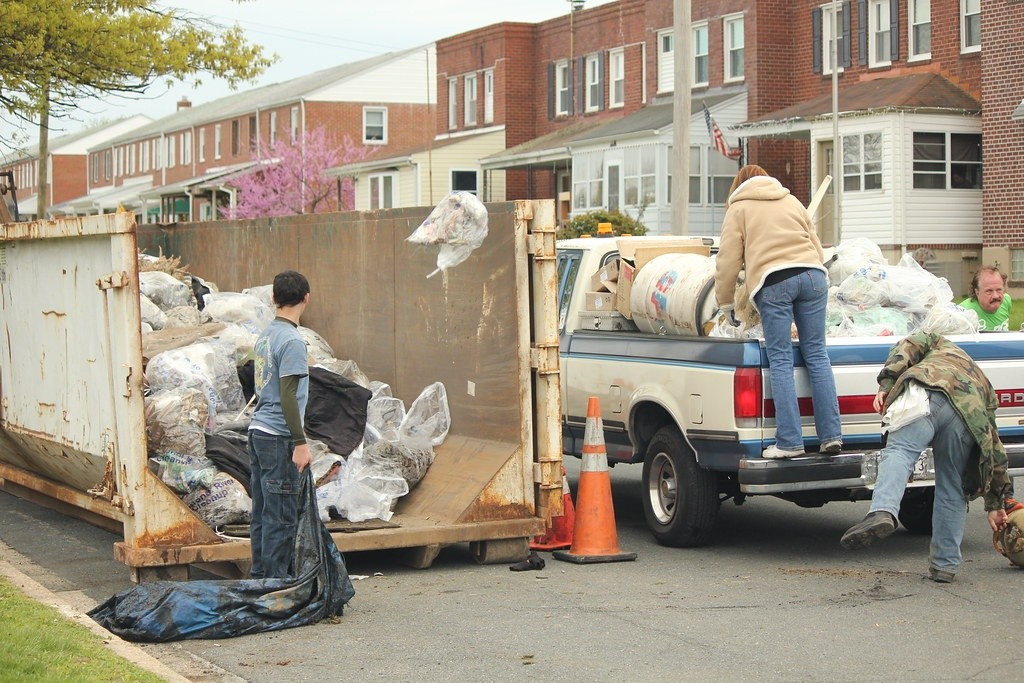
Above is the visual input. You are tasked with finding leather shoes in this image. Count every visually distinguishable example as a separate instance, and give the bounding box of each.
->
[929,567,955,583]
[840,511,894,551]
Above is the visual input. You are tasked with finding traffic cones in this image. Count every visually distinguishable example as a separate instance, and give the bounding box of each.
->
[528,462,579,552]
[554,396,639,563]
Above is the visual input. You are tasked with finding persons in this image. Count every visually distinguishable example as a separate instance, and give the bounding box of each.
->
[714,164,843,459]
[957,266,1013,331]
[248,270,313,579]
[839,330,1011,584]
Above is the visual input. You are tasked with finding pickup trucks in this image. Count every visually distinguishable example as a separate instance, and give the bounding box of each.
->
[551,222,1024,550]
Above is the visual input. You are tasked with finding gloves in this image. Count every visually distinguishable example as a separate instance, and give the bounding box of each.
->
[725,309,741,328]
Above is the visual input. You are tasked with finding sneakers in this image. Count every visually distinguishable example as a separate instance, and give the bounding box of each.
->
[763,445,805,458]
[820,439,843,452]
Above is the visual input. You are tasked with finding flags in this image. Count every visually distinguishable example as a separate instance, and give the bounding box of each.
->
[703,101,744,162]
